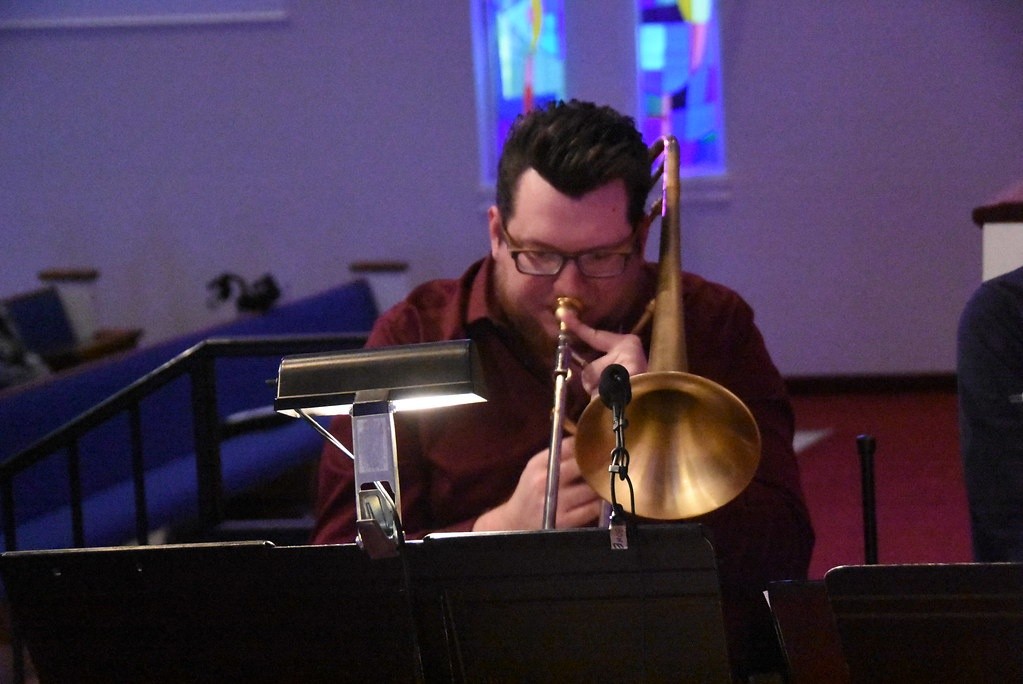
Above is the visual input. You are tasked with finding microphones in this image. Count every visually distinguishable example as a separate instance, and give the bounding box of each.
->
[597,365,633,523]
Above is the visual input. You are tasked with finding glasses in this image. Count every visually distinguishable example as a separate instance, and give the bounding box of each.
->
[500,218,642,279]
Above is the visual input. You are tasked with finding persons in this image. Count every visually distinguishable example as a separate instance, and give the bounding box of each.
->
[312,99,812,684]
[959,267,1023,564]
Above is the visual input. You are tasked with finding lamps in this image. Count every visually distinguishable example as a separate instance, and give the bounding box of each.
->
[269,337,490,557]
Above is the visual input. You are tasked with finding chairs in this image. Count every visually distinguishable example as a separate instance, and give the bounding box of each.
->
[0,288,78,370]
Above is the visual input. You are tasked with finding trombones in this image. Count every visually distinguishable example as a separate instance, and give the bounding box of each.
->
[542,134,764,534]
